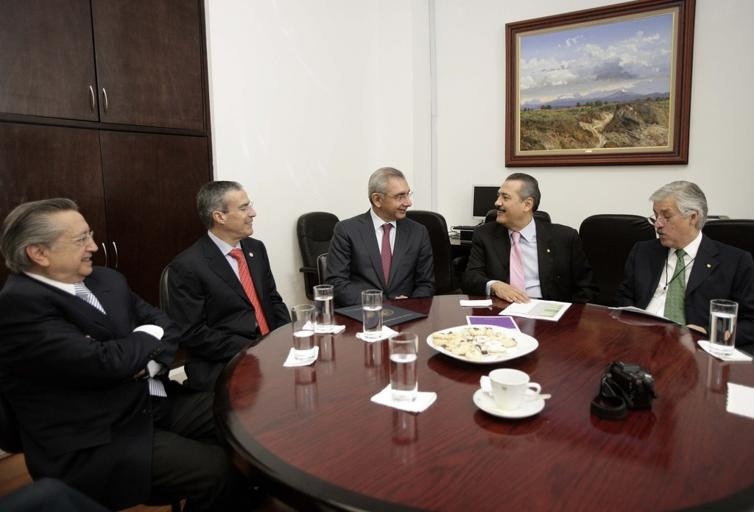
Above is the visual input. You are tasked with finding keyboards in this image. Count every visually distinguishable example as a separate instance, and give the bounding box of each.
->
[453,226,478,231]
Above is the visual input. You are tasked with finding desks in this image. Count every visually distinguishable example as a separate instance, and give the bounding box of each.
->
[447,234,473,291]
[211,296,754,512]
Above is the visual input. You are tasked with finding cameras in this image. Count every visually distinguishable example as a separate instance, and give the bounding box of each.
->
[607,361,656,412]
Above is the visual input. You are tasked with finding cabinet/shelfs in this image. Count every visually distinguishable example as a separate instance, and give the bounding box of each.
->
[1,0,210,134]
[0,122,214,303]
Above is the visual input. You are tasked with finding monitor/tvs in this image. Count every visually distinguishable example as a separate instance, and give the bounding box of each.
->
[473,187,501,216]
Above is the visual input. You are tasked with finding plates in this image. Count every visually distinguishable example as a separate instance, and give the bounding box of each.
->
[426,325,540,364]
[473,385,545,419]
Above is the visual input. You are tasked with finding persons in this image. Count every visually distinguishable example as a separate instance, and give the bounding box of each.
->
[456,172,595,304]
[612,180,752,350]
[317,166,436,308]
[163,180,290,365]
[0,194,237,511]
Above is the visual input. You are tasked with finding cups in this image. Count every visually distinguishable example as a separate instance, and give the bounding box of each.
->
[295,366,318,413]
[391,407,420,463]
[291,305,317,360]
[363,341,386,384]
[706,356,730,405]
[362,290,383,338]
[318,334,339,378]
[313,283,333,325]
[487,369,541,411]
[389,330,419,400]
[709,298,739,354]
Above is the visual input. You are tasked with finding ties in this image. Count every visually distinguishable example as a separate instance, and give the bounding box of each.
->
[664,249,685,326]
[229,248,270,337]
[512,232,525,292]
[74,281,167,398]
[381,224,391,286]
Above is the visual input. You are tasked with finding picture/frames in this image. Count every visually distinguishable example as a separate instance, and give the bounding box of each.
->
[504,0,696,169]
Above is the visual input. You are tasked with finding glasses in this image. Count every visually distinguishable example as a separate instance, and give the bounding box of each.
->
[377,190,413,201]
[648,208,699,227]
[221,200,254,214]
[55,230,94,247]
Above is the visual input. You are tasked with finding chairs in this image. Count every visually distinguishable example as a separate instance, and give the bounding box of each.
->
[406,211,465,295]
[159,260,202,364]
[297,211,341,304]
[700,218,754,256]
[1,382,184,512]
[580,213,656,306]
